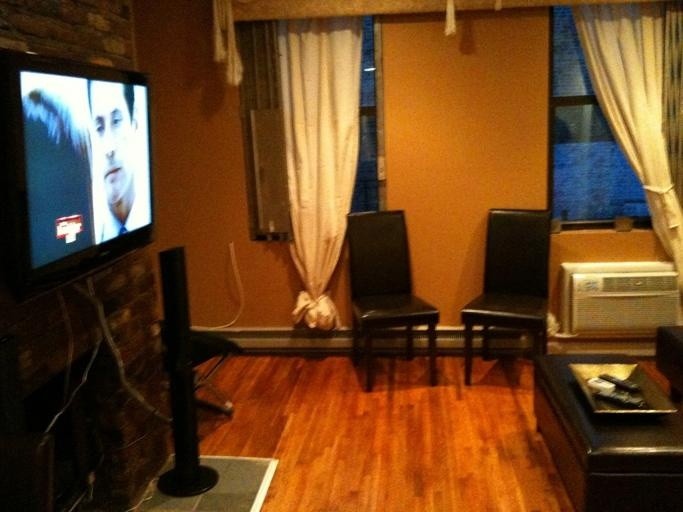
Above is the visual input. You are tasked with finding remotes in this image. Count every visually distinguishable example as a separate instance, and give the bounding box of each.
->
[587,377,615,391]
[592,390,646,409]
[599,373,640,392]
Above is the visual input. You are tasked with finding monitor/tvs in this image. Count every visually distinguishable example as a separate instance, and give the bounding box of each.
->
[1,47,156,308]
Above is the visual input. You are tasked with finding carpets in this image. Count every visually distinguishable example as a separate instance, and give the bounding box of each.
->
[125,451,278,511]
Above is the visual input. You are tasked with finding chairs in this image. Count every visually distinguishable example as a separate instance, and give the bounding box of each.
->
[344,208,553,391]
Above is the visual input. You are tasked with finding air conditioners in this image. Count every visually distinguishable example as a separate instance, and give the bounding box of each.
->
[554,262,681,341]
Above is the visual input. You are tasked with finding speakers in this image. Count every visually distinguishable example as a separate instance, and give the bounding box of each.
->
[157,244,217,495]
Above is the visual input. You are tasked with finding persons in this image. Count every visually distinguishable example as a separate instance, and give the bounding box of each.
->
[84,80,150,247]
[17,88,95,269]
